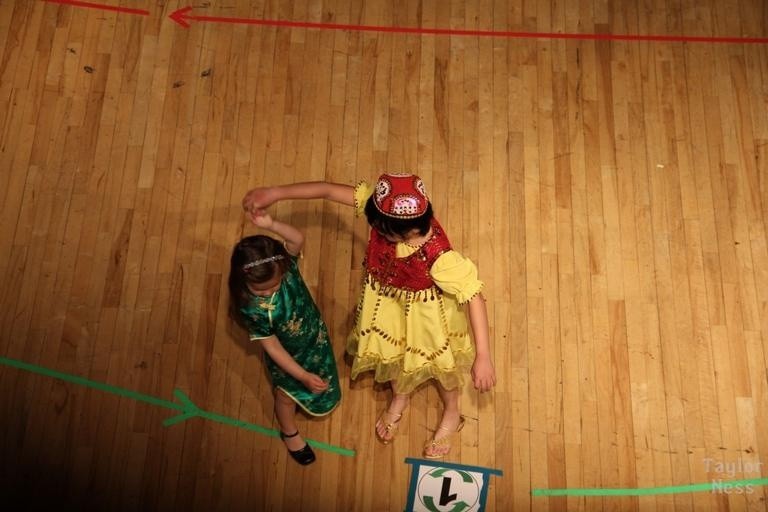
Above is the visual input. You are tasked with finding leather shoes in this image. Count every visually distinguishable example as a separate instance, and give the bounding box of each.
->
[280,429,315,465]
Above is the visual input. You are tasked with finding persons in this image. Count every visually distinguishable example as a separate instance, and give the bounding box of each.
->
[242,172,497,459]
[226,208,343,463]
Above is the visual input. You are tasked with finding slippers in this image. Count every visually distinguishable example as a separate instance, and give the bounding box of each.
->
[375,409,403,445]
[423,415,466,459]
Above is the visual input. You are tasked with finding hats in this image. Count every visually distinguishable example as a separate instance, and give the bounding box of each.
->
[372,170,429,220]
